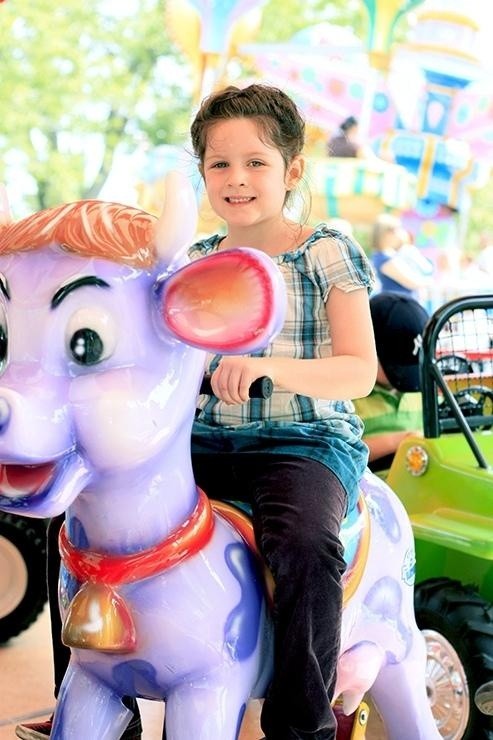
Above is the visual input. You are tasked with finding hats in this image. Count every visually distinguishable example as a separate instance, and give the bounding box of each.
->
[368,291,430,393]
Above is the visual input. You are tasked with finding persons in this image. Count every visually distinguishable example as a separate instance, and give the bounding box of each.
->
[326,117,383,166]
[163,83,378,739]
[344,291,445,472]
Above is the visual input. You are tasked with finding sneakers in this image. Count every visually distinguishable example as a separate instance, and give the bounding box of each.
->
[13,713,53,740]
